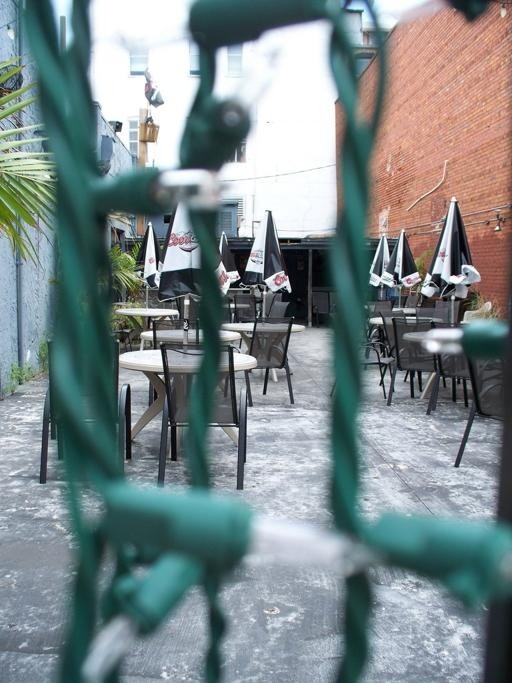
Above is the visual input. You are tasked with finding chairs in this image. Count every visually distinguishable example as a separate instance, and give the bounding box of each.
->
[312,289,336,328]
[368,298,504,465]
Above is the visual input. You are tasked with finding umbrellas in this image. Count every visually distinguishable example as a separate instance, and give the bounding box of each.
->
[422,197,481,328]
[158,196,231,353]
[244,205,294,324]
[370,231,393,301]
[133,220,162,326]
[218,229,240,299]
[385,224,421,307]
